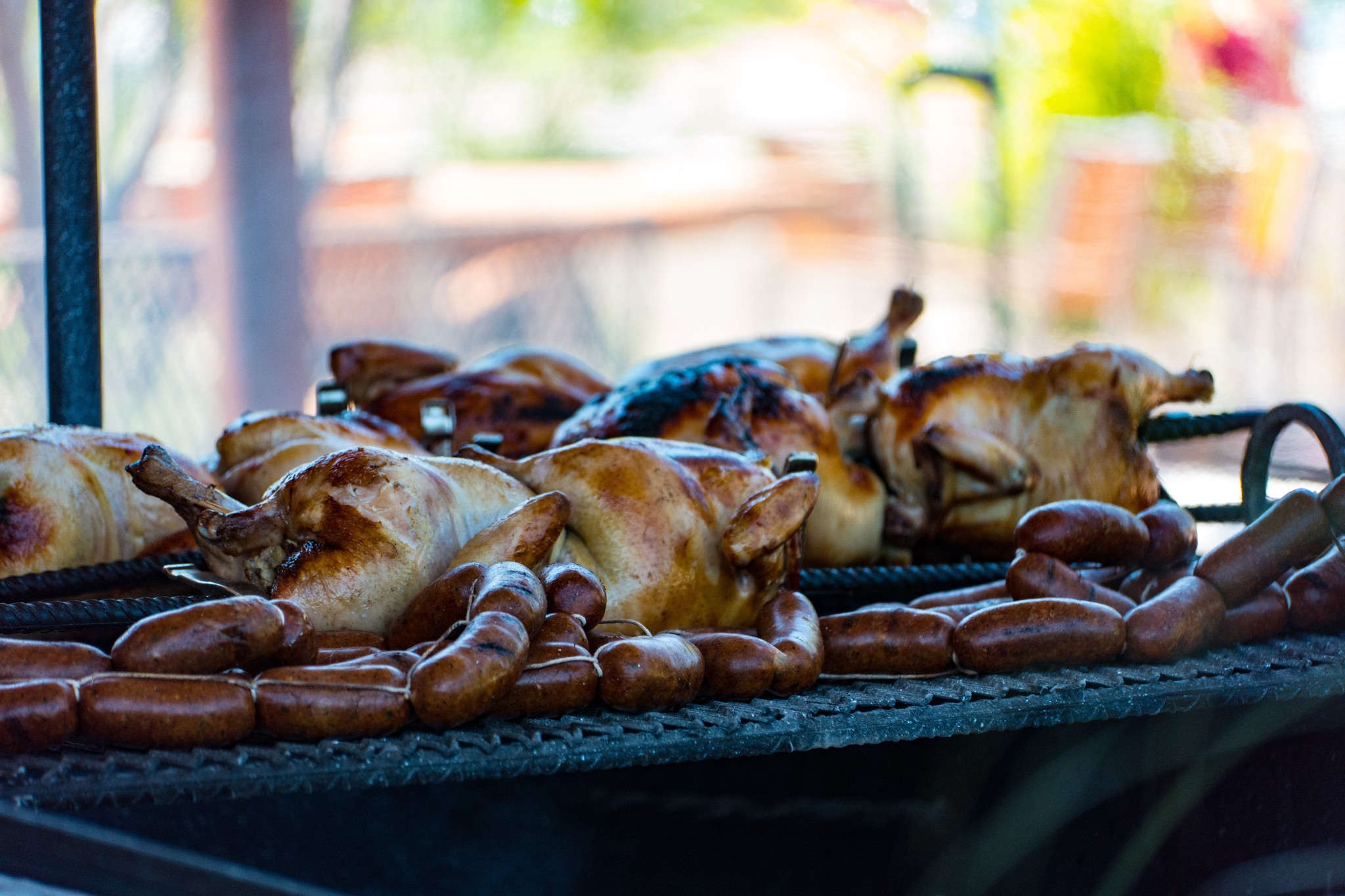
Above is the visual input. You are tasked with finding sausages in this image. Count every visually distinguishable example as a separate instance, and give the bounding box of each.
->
[0,468,1345,757]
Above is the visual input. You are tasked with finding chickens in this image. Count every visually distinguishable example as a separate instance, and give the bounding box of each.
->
[1,288,1215,635]
